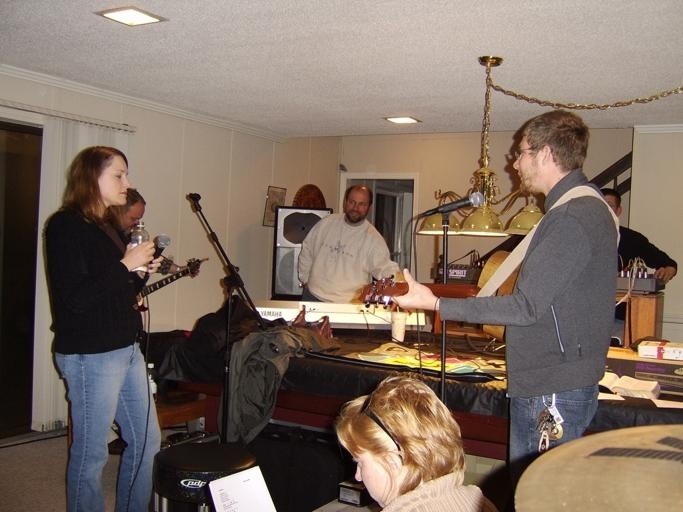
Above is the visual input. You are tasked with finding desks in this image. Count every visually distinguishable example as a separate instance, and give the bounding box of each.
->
[615,290,666,346]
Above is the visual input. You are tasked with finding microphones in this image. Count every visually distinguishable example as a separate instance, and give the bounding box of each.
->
[421,191,484,217]
[142,233,170,290]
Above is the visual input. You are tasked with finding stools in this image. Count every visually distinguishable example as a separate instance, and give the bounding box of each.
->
[152,443,258,510]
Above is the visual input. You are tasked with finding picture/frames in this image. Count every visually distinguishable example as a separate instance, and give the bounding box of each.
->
[269,205,331,302]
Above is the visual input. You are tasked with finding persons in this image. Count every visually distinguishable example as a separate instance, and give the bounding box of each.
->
[298,184,403,304]
[333,374,497,512]
[600,188,677,345]
[42,146,164,512]
[391,110,620,490]
[104,188,199,455]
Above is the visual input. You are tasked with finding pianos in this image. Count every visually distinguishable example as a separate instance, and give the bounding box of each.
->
[246,299,426,327]
[434,260,665,292]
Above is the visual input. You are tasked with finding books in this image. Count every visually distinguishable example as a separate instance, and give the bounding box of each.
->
[208,465,276,512]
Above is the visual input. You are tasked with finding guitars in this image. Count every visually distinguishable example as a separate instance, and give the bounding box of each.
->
[134,258,203,312]
[350,248,523,345]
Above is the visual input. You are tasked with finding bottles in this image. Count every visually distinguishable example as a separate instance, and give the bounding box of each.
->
[130,220,150,274]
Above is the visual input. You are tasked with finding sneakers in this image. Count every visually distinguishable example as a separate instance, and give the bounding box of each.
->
[106,439,128,454]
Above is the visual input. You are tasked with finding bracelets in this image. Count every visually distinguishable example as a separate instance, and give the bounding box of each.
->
[435,298,439,311]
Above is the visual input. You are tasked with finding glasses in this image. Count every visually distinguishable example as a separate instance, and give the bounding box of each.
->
[360,395,404,465]
[515,148,535,159]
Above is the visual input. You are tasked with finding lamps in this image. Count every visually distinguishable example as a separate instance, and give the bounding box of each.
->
[418,54,547,233]
